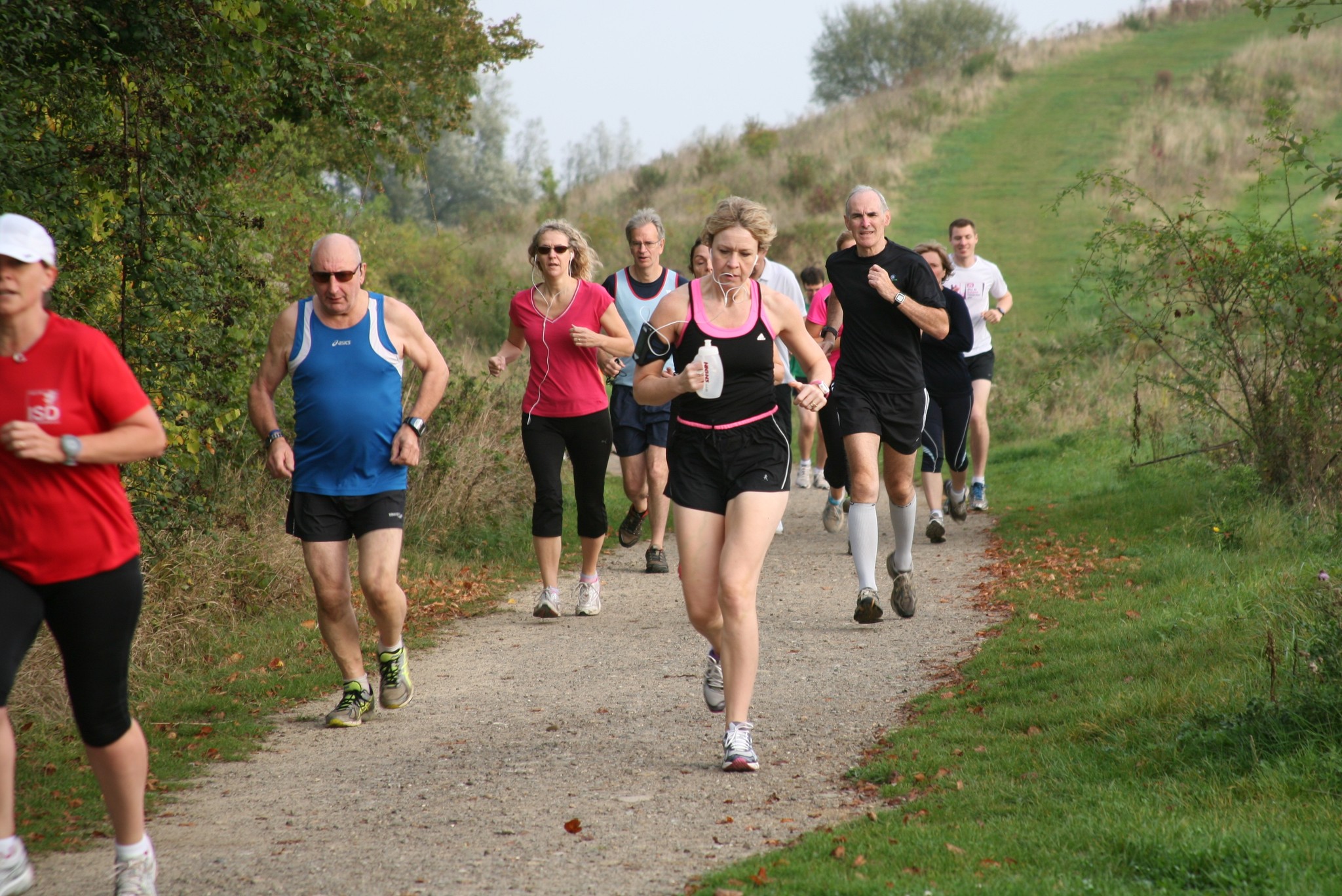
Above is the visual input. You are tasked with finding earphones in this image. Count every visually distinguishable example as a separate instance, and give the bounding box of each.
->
[535,254,538,261]
[753,255,759,266]
[570,252,573,261]
[709,249,712,258]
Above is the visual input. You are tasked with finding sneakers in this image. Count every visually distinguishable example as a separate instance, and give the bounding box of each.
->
[376,645,415,709]
[853,588,883,623]
[796,462,812,490]
[925,513,947,544]
[944,479,967,525]
[812,472,830,489]
[325,680,376,728]
[533,590,561,618]
[113,842,158,896]
[645,544,669,573]
[720,721,760,770]
[575,573,601,616]
[887,550,918,618]
[822,486,846,533]
[618,494,650,548]
[967,482,989,511]
[703,646,726,713]
[0,837,34,896]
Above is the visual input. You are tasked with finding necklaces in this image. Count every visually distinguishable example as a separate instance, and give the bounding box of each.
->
[0,312,45,363]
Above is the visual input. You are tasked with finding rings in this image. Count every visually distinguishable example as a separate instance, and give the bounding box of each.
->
[696,368,702,375]
[810,401,818,408]
[577,338,581,342]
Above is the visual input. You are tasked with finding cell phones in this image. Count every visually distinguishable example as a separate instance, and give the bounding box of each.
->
[632,322,652,366]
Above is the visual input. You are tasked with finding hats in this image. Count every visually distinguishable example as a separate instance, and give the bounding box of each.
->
[0,213,57,266]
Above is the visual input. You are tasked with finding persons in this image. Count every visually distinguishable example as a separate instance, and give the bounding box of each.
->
[748,242,809,533]
[631,194,833,771]
[591,207,693,572]
[908,243,976,543]
[813,183,951,622]
[687,236,786,384]
[787,267,831,490]
[488,222,635,619]
[1,210,171,895]
[934,217,1014,515]
[803,229,860,538]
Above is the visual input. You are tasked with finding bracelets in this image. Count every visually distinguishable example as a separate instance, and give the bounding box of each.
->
[821,326,838,341]
[995,306,1005,317]
[263,427,282,449]
[246,232,450,729]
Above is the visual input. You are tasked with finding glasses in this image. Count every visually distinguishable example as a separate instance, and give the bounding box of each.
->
[535,245,572,254]
[310,262,362,282]
[629,239,661,248]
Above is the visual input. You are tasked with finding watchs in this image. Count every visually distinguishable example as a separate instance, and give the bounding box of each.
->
[402,416,426,437]
[892,291,906,309]
[809,380,830,398]
[57,430,84,468]
